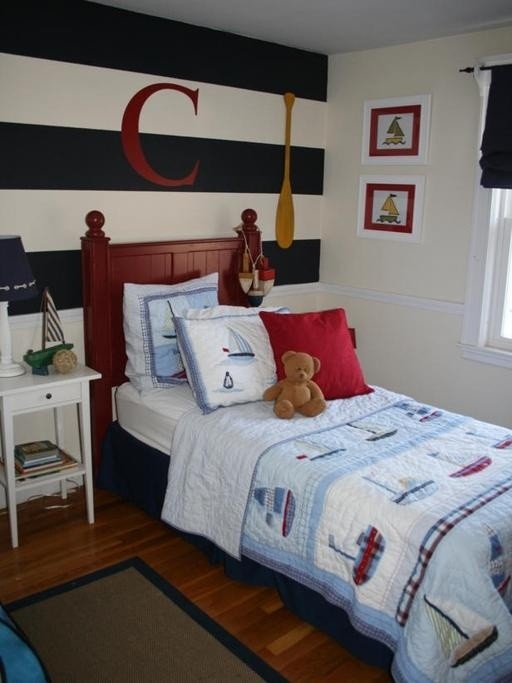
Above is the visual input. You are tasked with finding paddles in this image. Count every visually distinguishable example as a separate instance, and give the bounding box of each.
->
[276,93,295,248]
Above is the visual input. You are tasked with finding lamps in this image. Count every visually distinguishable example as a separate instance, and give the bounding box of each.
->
[0,235,39,380]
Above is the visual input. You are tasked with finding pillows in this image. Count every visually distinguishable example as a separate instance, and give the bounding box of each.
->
[171,305,290,415]
[258,308,375,400]
[127,272,219,399]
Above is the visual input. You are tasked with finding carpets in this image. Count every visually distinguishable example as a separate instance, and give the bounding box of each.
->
[6,555,293,682]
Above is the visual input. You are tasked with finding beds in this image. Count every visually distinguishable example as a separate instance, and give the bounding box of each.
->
[78,209,512,683]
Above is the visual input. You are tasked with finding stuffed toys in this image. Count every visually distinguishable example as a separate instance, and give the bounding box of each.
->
[262,349,327,419]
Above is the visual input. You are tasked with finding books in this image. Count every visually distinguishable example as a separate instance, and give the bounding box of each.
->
[0,438,82,489]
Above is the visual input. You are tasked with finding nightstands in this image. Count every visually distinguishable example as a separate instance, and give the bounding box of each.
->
[0,363,101,548]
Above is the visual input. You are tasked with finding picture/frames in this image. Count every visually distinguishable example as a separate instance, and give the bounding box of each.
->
[357,175,425,243]
[362,94,431,165]
